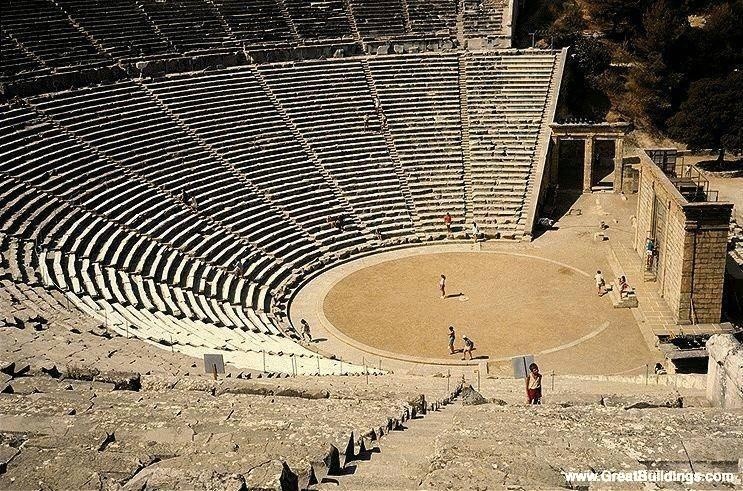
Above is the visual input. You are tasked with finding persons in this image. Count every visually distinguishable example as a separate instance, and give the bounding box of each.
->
[231,256,245,280]
[460,335,473,361]
[593,271,606,295]
[446,326,455,355]
[362,114,369,133]
[300,320,311,342]
[439,275,447,300]
[618,277,626,299]
[523,363,542,405]
[190,194,197,213]
[683,163,691,179]
[180,189,189,207]
[443,213,452,234]
[472,221,478,242]
[374,228,383,241]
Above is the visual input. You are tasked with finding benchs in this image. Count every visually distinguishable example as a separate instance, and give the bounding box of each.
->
[1,0,743,490]
